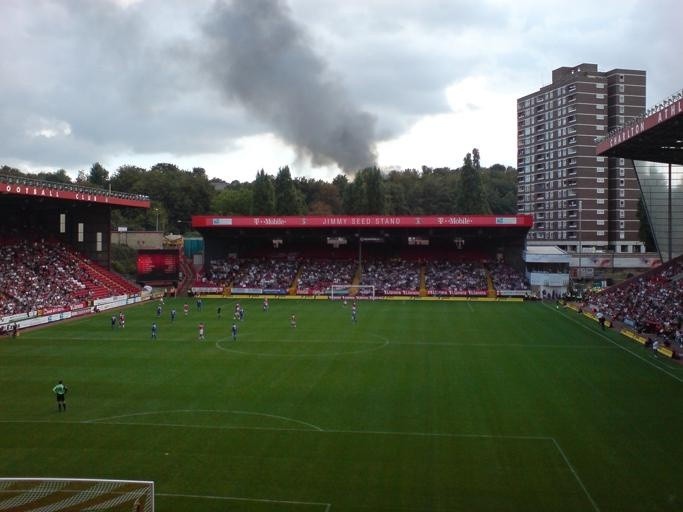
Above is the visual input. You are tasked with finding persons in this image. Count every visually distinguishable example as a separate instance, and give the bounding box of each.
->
[111,311,126,329]
[150,296,208,342]
[289,313,297,328]
[216,299,268,340]
[351,302,357,323]
[52,380,68,411]
[0,235,99,338]
[538,255,683,359]
[201,236,530,298]
